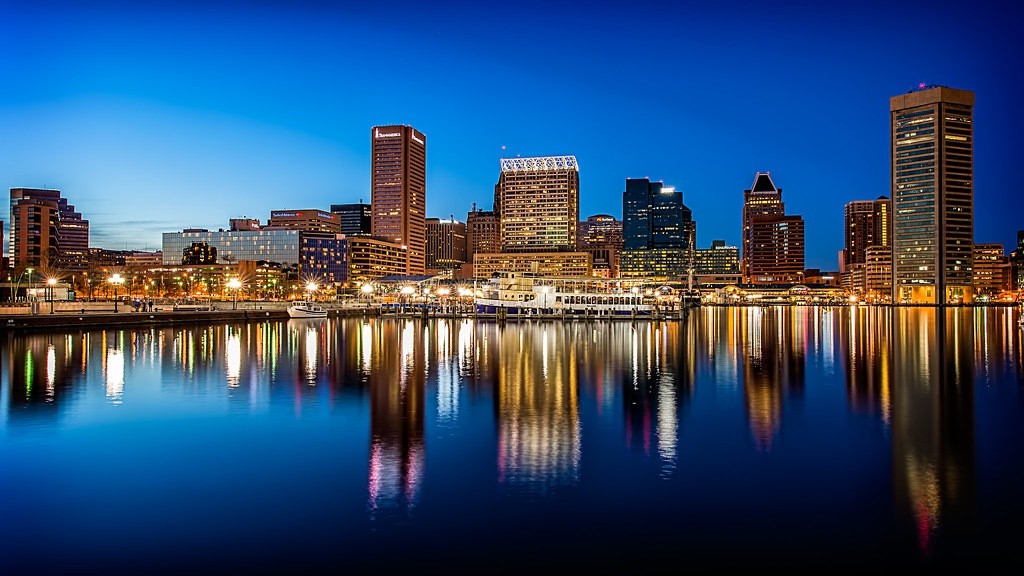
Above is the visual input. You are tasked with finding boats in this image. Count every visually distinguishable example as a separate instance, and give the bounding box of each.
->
[284,303,329,319]
[651,285,685,320]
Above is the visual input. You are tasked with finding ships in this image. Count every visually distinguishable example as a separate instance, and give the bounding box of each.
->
[471,281,660,320]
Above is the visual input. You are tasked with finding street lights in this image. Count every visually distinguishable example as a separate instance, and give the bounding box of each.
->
[227,278,242,309]
[306,282,318,308]
[438,288,450,307]
[48,278,58,315]
[361,285,373,309]
[402,288,414,307]
[459,287,467,308]
[107,272,125,314]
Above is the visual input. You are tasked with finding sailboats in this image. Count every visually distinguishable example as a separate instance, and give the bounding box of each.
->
[681,234,701,309]
